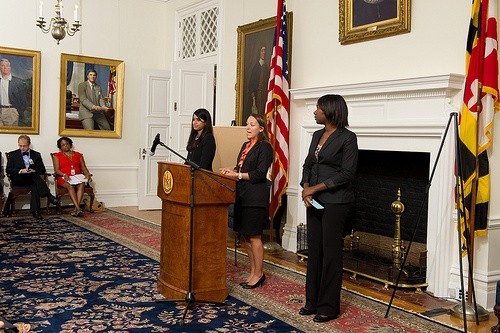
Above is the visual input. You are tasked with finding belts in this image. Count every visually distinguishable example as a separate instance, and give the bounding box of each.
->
[0,105,16,108]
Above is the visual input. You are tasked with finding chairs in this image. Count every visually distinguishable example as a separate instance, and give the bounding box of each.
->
[50,153,95,213]
[228,193,287,249]
[0,152,7,215]
[5,152,50,215]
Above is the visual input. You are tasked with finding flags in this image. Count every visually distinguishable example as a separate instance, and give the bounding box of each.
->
[264,0,291,219]
[454,0,500,256]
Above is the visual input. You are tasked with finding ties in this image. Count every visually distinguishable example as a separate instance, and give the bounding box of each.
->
[92,85,96,105]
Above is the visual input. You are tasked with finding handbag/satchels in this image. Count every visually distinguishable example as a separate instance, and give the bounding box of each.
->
[82,198,106,212]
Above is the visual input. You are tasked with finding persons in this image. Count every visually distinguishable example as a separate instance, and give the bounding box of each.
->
[78,70,113,130]
[53,137,92,216]
[220,113,273,289]
[0,58,28,126]
[185,108,216,172]
[299,94,358,323]
[6,135,59,219]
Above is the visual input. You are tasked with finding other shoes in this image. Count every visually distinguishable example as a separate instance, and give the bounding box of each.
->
[299,307,316,315]
[70,209,78,216]
[77,208,84,216]
[313,314,330,321]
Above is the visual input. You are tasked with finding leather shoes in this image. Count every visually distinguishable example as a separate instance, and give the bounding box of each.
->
[33,211,42,219]
[48,195,61,206]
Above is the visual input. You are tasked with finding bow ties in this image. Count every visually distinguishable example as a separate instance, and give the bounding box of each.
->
[23,152,29,156]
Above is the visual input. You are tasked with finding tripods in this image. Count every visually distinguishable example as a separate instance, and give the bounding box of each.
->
[153,139,236,327]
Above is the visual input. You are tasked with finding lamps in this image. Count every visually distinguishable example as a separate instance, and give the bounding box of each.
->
[35,0,82,46]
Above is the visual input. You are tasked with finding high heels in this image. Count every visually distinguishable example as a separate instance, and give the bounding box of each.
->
[240,280,249,286]
[245,275,266,288]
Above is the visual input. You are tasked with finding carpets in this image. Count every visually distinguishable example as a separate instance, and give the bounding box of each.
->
[0,205,472,333]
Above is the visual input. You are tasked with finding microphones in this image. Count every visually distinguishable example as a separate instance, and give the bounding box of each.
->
[151,134,160,152]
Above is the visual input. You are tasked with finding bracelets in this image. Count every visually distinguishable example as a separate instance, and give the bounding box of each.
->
[239,173,242,179]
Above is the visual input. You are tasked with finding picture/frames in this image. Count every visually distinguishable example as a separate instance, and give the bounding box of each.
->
[0,46,41,135]
[234,11,294,127]
[338,0,411,46]
[58,53,126,140]
